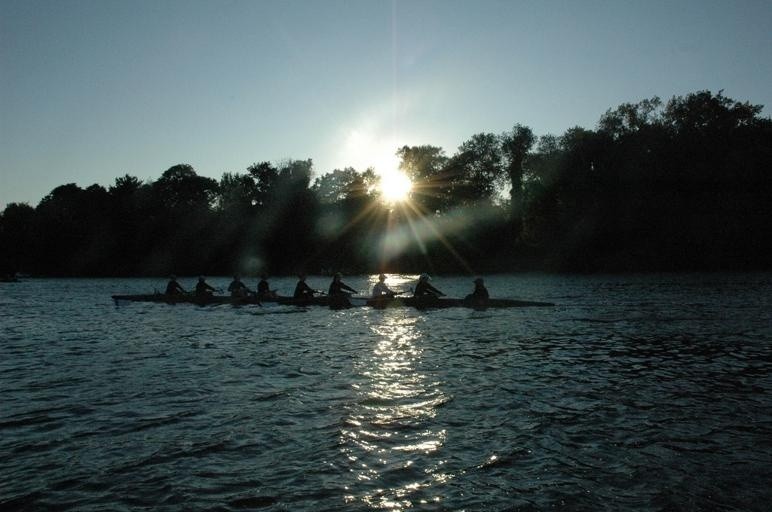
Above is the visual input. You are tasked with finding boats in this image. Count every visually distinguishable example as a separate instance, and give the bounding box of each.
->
[110,292,558,309]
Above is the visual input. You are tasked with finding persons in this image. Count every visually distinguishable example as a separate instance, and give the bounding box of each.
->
[372,273,402,297]
[414,272,447,296]
[327,272,357,294]
[463,278,489,299]
[256,271,270,294]
[293,269,318,297]
[227,273,257,295]
[166,274,188,294]
[196,276,219,295]
